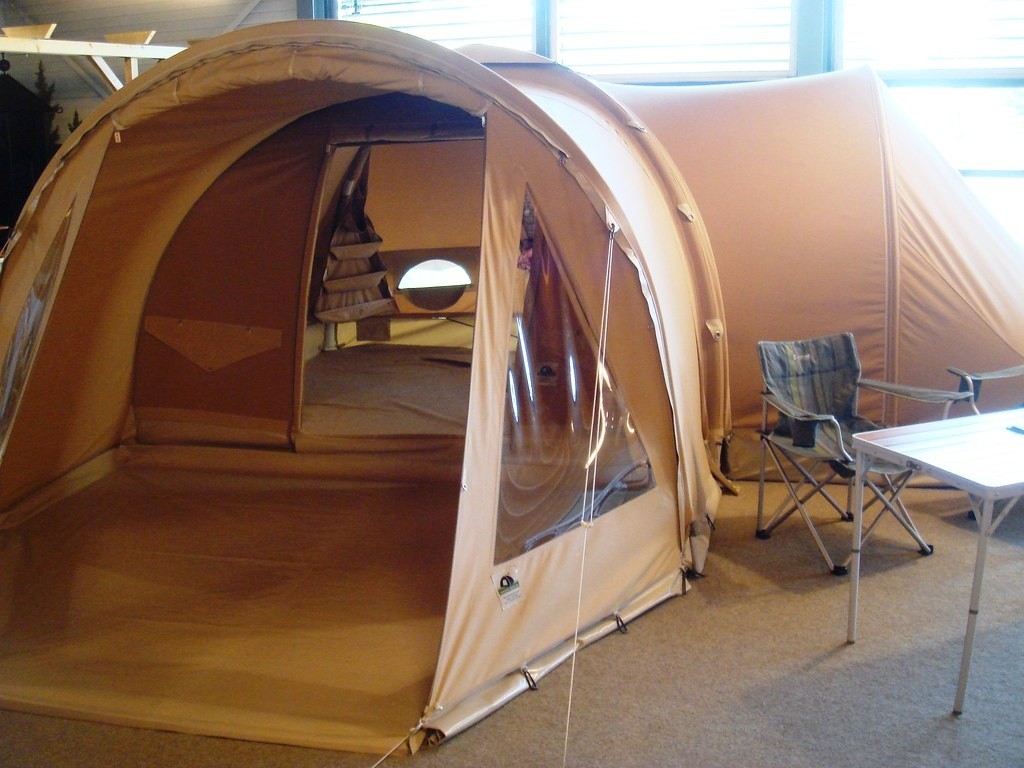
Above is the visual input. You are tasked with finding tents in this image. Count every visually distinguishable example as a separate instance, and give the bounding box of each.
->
[296,68,1024,493]
[2,19,730,756]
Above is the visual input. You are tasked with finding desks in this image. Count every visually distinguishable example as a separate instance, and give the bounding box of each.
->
[846,407,1023,714]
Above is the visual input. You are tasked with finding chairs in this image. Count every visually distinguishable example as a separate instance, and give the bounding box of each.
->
[756,332,973,575]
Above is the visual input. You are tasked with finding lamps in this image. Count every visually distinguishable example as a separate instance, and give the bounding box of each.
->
[398,258,472,311]
